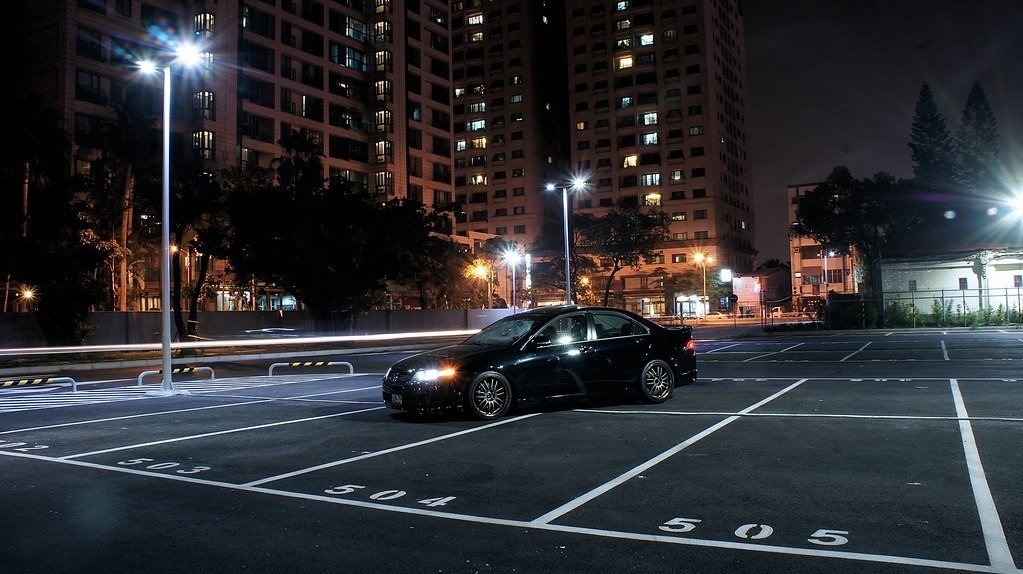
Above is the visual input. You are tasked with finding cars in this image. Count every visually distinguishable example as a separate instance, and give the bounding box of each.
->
[381,305,701,422]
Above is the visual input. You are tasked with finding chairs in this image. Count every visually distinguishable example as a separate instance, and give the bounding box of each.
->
[571,324,586,342]
[543,326,557,343]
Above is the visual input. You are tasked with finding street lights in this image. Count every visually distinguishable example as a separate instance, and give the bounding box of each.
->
[140,44,200,389]
[545,179,587,336]
[694,252,714,320]
[821,252,835,307]
[504,254,522,316]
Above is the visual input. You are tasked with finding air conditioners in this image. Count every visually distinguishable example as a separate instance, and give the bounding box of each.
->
[792,286,802,295]
[793,248,799,253]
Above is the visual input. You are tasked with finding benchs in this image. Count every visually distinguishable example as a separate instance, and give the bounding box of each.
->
[595,323,631,339]
